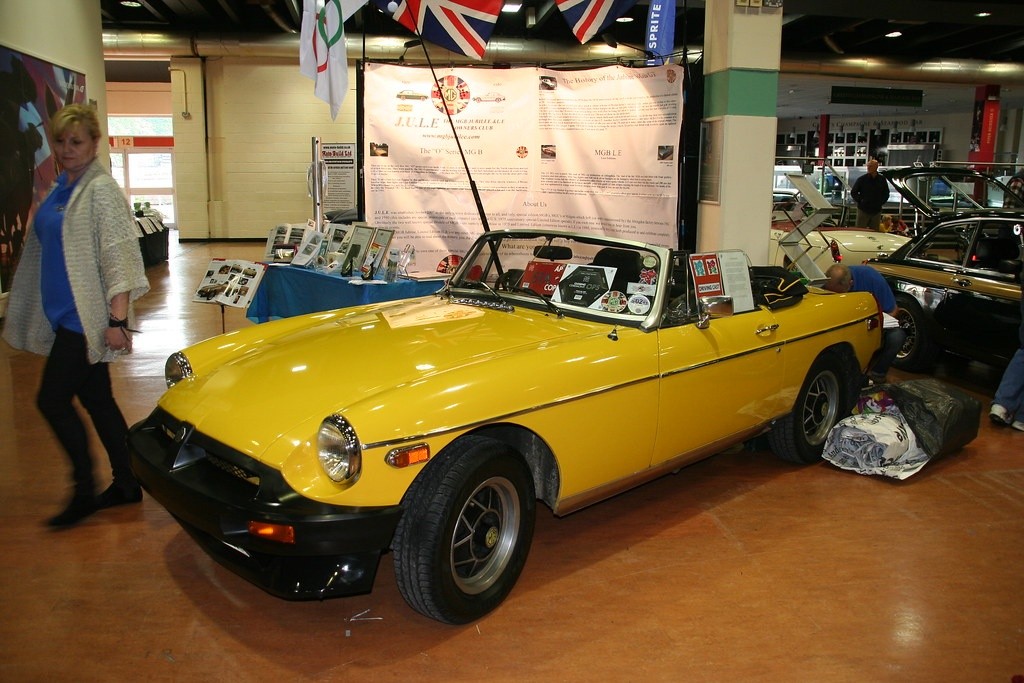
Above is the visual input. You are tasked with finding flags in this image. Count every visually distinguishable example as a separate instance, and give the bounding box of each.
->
[299,0,640,123]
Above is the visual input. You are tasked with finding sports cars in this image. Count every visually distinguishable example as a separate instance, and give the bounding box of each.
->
[125,229,890,630]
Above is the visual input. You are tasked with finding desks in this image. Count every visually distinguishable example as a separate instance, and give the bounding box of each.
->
[246,261,448,325]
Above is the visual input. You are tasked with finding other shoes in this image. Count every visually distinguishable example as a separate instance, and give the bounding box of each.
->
[98,473,143,509]
[43,476,100,531]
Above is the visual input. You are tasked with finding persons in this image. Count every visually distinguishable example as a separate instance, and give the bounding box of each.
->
[3,105,150,533]
[819,264,905,386]
[852,160,890,231]
[1004,168,1024,209]
[989,326,1024,432]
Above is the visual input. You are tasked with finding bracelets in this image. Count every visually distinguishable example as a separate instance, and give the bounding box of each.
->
[108,313,143,341]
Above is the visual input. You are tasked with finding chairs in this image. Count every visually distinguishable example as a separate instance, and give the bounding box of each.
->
[974,238,1024,277]
[593,248,643,297]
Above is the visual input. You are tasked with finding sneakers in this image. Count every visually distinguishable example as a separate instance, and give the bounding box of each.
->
[988,404,1013,426]
[1012,421,1024,431]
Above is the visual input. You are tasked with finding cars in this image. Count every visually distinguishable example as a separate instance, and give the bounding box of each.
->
[853,216,1024,368]
[770,161,1014,290]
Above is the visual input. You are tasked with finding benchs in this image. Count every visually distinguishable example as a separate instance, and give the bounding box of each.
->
[671,266,808,313]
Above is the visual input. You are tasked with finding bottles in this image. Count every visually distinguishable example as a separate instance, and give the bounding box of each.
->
[385,248,398,282]
[397,244,411,266]
[398,249,415,273]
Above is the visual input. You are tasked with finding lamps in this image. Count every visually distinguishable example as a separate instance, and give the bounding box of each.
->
[813,125,823,138]
[859,125,866,137]
[838,126,847,137]
[873,124,882,135]
[790,128,798,138]
[891,122,899,134]
[909,119,916,132]
